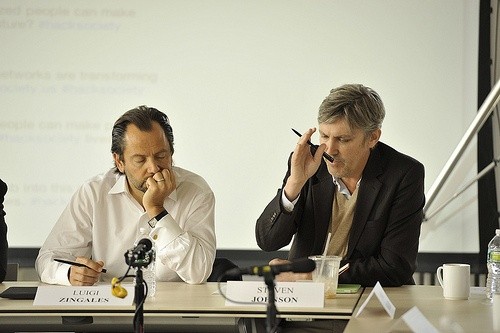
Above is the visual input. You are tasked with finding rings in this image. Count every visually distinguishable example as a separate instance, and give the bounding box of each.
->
[156,179,164,182]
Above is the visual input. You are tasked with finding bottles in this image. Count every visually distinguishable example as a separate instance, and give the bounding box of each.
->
[486,229,500,299]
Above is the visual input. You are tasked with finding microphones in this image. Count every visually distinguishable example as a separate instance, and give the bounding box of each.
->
[226,257,316,276]
[128,238,152,263]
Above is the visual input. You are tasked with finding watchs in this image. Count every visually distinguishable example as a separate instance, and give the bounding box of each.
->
[146,208,169,229]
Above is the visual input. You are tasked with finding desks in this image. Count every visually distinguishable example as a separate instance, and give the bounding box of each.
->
[0,280,499,333]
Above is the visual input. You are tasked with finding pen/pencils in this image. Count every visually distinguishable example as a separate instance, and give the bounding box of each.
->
[54,259,106,274]
[291,128,334,164]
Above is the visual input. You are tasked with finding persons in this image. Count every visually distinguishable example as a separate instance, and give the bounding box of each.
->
[253,84,427,288]
[34,103,218,287]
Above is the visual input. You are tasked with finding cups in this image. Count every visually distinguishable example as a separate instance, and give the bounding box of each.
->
[490,293,500,330]
[308,255,342,298]
[437,263,471,300]
